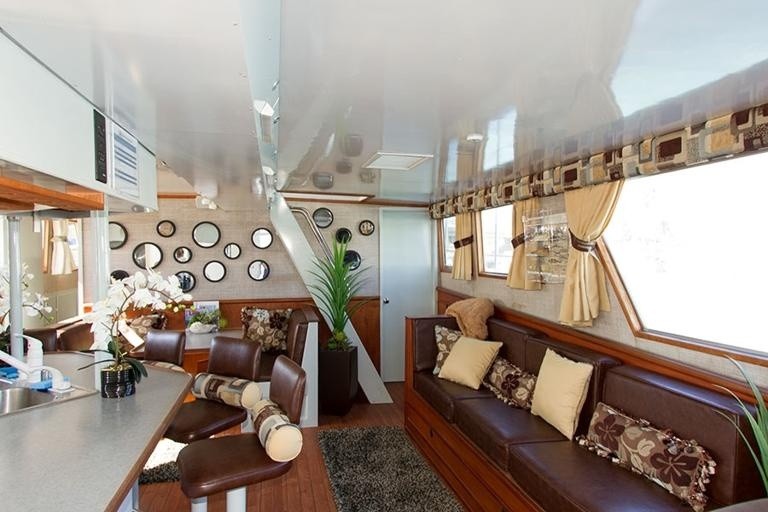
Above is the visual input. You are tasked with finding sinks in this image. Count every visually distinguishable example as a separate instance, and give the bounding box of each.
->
[1,383,97,415]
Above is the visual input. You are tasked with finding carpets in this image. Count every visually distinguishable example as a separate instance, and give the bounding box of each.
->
[317,425,463,511]
[137,435,214,485]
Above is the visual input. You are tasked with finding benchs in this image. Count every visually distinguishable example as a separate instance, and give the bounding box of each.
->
[239,304,319,433]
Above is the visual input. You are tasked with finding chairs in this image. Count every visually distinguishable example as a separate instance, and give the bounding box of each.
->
[22,328,57,352]
[144,330,306,512]
[60,323,94,351]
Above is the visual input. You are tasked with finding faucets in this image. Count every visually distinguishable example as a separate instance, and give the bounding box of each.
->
[0,331,43,383]
[29,365,75,393]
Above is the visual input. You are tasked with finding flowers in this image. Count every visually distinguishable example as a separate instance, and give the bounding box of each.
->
[0,263,55,345]
[185,305,228,329]
[78,269,196,384]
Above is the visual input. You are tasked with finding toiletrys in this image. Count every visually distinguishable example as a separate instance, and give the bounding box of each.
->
[29,376,69,390]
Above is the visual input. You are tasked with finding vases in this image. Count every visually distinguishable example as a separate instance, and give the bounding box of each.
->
[100,367,135,398]
[188,322,216,333]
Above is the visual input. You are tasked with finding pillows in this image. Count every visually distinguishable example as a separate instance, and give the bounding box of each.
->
[531,348,595,441]
[240,305,293,353]
[433,325,463,375]
[445,298,495,339]
[481,355,537,409]
[120,312,168,353]
[576,402,717,511]
[437,335,503,389]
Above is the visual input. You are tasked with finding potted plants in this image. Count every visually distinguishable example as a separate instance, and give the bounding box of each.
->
[305,234,374,417]
[0,344,10,366]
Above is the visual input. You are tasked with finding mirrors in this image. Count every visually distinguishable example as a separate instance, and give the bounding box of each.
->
[312,207,375,271]
[107,220,273,293]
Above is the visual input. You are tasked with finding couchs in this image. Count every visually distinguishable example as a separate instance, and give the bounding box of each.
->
[405,307,768,511]
